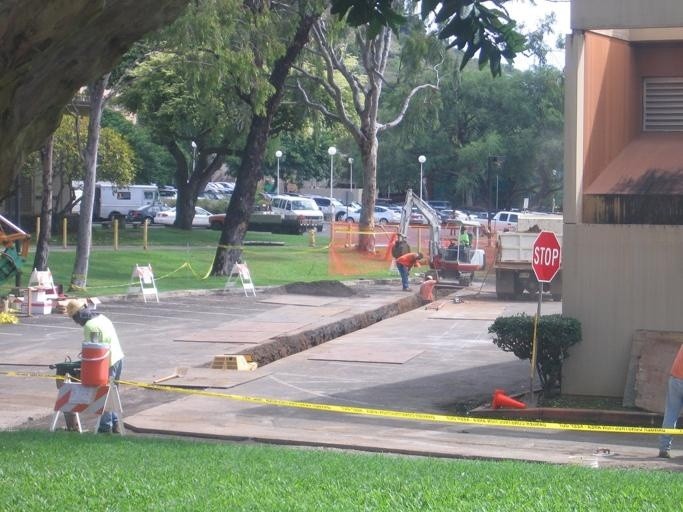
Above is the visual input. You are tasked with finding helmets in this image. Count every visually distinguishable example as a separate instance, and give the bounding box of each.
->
[66,299,84,316]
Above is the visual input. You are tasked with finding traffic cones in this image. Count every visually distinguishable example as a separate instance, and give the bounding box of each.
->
[492,390,526,409]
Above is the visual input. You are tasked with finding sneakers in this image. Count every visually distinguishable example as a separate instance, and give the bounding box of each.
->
[98,422,121,433]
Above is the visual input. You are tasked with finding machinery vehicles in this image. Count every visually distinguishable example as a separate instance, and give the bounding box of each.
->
[0,215,31,281]
[390,189,493,286]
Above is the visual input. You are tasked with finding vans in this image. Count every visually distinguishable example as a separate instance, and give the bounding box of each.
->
[94,183,160,228]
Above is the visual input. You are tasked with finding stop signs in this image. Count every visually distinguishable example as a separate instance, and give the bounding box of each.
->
[532,231,561,283]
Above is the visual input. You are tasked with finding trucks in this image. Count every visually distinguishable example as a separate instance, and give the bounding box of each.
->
[493,213,563,295]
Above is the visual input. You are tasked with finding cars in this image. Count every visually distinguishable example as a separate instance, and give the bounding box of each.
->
[160,181,236,199]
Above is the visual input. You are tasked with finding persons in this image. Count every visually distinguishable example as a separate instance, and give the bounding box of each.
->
[394,251,423,292]
[657,342,682,458]
[66,299,126,434]
[419,275,438,306]
[449,227,470,248]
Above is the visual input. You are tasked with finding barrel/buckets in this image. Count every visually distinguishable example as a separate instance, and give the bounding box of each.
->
[77,342,110,385]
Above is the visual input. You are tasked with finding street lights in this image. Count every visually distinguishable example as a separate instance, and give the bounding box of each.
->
[328,146,336,198]
[418,155,427,199]
[275,150,283,194]
[487,155,504,246]
[348,157,354,191]
[191,142,197,172]
